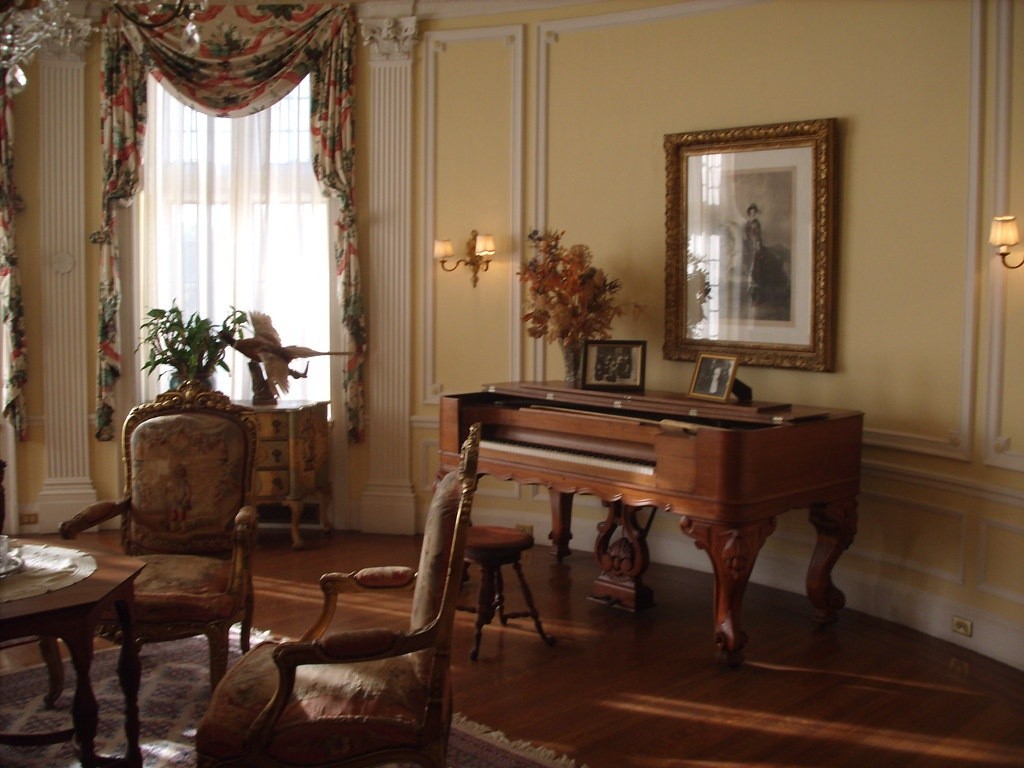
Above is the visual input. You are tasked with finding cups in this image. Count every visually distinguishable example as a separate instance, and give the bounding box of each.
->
[0,535,9,567]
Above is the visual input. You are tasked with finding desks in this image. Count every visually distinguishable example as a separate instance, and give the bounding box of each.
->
[0,534,147,768]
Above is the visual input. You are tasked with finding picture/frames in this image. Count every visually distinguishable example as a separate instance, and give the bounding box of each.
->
[580,339,646,392]
[688,352,740,403]
[661,115,833,372]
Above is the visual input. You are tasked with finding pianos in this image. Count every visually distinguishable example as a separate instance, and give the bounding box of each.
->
[434,377,867,669]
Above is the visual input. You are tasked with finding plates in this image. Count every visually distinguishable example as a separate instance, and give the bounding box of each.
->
[0,554,23,578]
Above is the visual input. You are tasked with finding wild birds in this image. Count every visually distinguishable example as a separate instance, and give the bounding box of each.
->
[216,312,356,395]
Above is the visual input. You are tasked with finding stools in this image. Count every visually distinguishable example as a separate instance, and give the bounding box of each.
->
[458,528,554,663]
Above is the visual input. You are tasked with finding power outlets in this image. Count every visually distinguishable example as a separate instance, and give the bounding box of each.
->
[951,616,972,638]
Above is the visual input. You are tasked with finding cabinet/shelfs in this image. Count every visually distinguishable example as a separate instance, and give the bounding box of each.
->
[252,400,332,549]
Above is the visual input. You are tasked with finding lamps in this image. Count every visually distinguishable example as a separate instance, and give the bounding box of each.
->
[989,213,1024,279]
[432,228,496,289]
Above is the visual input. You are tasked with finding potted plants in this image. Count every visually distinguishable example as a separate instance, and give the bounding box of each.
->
[515,230,625,389]
[135,307,248,390]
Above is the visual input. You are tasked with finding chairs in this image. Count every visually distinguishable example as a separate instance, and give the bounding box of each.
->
[194,418,482,768]
[40,379,254,713]
